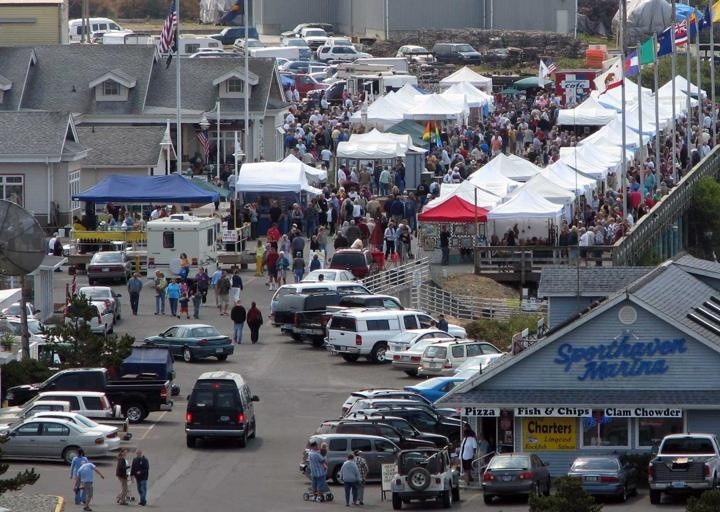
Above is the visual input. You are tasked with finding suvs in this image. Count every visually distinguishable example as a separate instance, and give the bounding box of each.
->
[329,247,381,288]
[316,387,465,456]
[418,342,503,377]
[273,292,347,345]
[1,390,122,420]
[301,432,403,485]
[390,445,460,510]
[323,310,466,364]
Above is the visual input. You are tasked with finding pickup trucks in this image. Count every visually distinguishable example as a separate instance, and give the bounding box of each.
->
[648,432,719,505]
[293,294,406,350]
[1,400,132,442]
[6,366,173,423]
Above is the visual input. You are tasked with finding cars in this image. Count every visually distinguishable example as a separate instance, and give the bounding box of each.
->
[0,411,121,452]
[454,366,486,384]
[144,324,234,363]
[567,454,639,503]
[0,417,108,467]
[573,41,587,59]
[404,376,466,402]
[384,329,475,361]
[0,285,122,365]
[453,352,509,375]
[481,453,553,507]
[390,338,464,376]
[88,251,132,286]
[299,268,363,285]
[487,48,508,61]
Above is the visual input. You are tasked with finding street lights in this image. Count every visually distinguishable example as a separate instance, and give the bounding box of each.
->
[230,130,246,251]
[160,118,172,175]
[360,91,371,134]
[330,129,341,191]
[200,101,221,180]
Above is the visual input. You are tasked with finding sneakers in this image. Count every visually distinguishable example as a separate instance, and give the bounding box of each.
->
[84,507,92,511]
[312,495,323,502]
[358,498,363,505]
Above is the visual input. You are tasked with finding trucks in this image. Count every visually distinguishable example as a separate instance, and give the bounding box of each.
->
[147,214,222,282]
[110,241,147,266]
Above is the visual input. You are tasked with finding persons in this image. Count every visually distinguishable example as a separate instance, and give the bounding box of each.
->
[72,460,104,512]
[130,449,149,506]
[306,440,333,501]
[340,454,361,508]
[352,448,370,505]
[69,449,88,506]
[460,423,489,486]
[115,447,130,505]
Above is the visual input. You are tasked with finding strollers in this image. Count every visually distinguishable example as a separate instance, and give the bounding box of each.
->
[303,467,338,503]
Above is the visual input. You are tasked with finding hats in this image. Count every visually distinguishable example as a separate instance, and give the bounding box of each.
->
[356,450,362,455]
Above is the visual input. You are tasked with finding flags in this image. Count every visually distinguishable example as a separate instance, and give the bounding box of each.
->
[151,1,177,64]
[163,34,177,70]
[593,1,720,96]
[213,1,244,27]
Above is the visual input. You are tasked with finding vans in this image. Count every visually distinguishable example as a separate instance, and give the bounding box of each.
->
[268,281,375,326]
[432,42,482,65]
[700,42,720,61]
[184,370,260,451]
